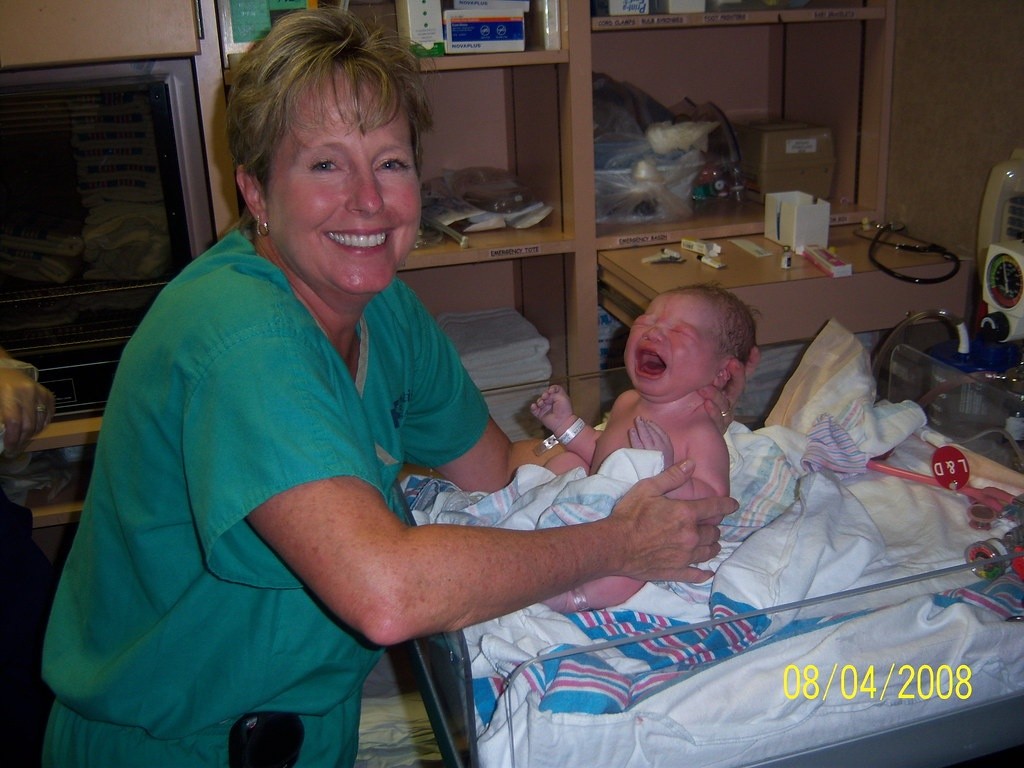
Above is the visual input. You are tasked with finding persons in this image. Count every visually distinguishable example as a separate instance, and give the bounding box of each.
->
[40,9,759,768]
[0,346,57,461]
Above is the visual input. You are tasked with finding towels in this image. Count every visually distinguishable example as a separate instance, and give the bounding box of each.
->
[385,413,1024,766]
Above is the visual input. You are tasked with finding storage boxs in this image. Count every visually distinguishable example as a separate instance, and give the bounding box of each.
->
[650,0,705,14]
[765,190,832,254]
[731,119,836,205]
[590,0,649,17]
[405,0,445,58]
[442,8,526,54]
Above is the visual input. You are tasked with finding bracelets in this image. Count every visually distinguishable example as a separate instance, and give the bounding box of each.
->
[532,417,587,458]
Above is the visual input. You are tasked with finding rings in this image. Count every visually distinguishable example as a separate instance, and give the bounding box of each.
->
[34,404,49,414]
[720,399,734,417]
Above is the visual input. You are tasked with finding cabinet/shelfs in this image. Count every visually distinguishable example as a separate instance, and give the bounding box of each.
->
[0,60,217,530]
[194,0,976,434]
